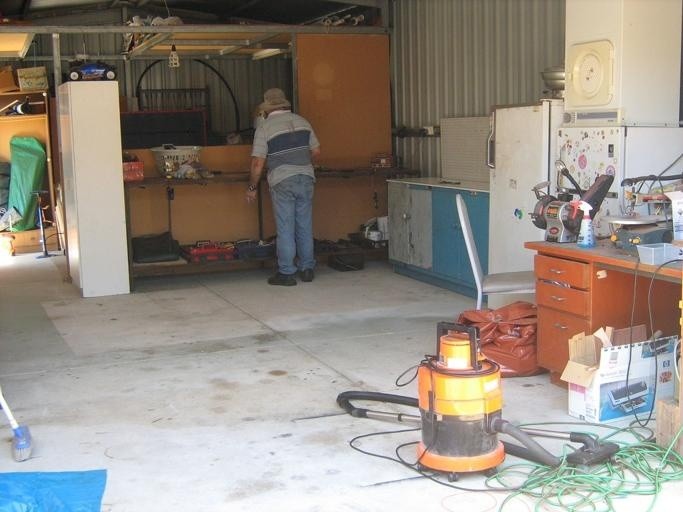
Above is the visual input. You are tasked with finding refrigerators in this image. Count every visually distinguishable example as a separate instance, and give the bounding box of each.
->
[487,100,562,312]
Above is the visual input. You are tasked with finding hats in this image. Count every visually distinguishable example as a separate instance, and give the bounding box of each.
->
[256,87,291,111]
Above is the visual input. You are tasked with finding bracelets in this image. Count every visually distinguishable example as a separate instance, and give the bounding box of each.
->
[247,183,258,191]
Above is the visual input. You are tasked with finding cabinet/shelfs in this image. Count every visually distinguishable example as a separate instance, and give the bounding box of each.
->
[57,79,130,298]
[384,175,489,304]
[0,88,59,255]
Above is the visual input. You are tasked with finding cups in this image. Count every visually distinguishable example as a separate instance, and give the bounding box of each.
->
[376,217,387,241]
[369,230,381,249]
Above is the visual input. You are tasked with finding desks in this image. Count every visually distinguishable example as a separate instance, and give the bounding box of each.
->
[523,236,682,391]
[127,166,392,277]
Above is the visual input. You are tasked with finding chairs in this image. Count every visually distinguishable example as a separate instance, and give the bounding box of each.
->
[455,192,537,312]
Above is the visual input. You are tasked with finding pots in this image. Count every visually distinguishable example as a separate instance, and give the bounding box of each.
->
[539,68,564,99]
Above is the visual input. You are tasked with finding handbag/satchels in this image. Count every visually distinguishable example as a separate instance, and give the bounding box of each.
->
[327,253,365,272]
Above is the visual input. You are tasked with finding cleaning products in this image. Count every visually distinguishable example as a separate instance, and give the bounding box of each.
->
[569,199,597,249]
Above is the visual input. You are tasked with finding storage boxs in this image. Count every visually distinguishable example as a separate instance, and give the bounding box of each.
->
[665,188,682,242]
[635,239,680,265]
[559,323,678,425]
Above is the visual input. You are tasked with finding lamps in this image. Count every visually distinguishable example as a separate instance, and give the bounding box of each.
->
[167,32,181,73]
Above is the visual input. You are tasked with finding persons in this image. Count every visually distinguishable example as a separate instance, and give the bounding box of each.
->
[243,87,321,286]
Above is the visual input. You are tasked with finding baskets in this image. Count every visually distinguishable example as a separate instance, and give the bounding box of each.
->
[151,143,202,177]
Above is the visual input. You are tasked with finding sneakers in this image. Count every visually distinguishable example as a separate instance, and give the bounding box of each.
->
[299,268,314,282]
[267,272,296,286]
